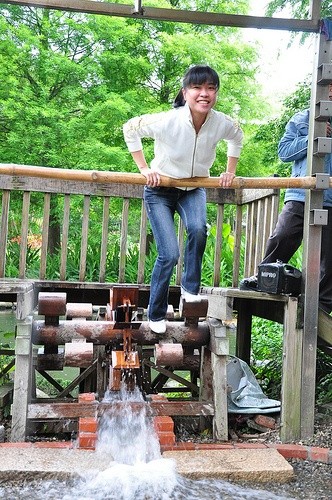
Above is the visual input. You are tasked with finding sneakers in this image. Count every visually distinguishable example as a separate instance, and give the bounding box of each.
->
[181,286,201,304]
[147,304,166,334]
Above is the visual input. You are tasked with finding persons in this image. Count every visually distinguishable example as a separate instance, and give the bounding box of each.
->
[241,79,332,318]
[123,67,245,334]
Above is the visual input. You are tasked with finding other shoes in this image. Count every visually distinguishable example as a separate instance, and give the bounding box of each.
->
[240,273,260,290]
